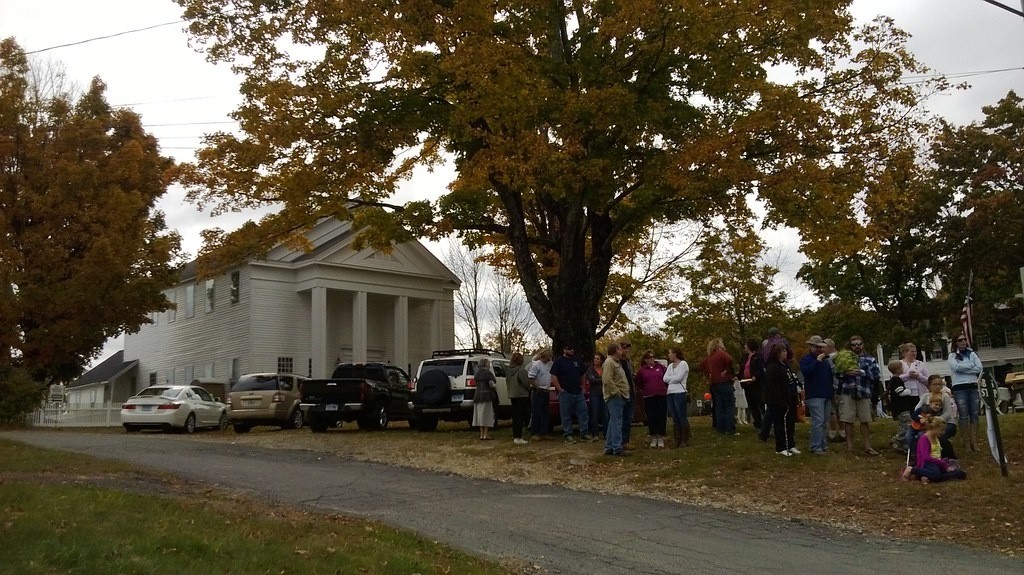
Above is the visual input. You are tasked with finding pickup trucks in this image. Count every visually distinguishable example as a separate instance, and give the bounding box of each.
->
[301,358,415,431]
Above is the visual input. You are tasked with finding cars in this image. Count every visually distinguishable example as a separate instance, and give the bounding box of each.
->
[119,384,230,433]
[535,366,650,430]
[230,372,314,430]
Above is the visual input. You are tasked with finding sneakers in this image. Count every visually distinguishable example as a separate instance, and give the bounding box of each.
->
[789,447,801,454]
[775,449,792,457]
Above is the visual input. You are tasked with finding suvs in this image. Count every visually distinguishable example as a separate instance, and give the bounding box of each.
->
[406,348,522,432]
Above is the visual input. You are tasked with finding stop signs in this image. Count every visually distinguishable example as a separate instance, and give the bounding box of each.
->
[976,372,1002,409]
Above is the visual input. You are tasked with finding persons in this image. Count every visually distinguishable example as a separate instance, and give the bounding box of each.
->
[731,323,893,428]
[942,360,1017,417]
[947,333,983,453]
[837,334,881,458]
[763,342,802,457]
[898,343,930,418]
[905,392,943,462]
[799,335,835,456]
[662,346,692,449]
[506,353,531,445]
[585,352,608,441]
[904,375,959,462]
[887,360,916,454]
[634,349,669,448]
[471,359,497,440]
[703,338,740,436]
[601,341,632,457]
[550,342,595,444]
[528,349,556,441]
[618,342,636,450]
[900,415,967,484]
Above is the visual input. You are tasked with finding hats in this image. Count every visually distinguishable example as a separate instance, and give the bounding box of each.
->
[823,337,834,346]
[769,327,783,333]
[619,342,632,348]
[807,335,826,347]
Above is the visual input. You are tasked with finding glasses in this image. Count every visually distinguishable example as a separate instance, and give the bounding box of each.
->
[566,347,575,351]
[851,342,863,346]
[957,338,968,342]
[647,356,656,359]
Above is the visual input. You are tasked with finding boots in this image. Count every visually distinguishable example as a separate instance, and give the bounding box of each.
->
[961,424,970,453]
[970,423,982,452]
[681,423,690,448]
[670,423,680,448]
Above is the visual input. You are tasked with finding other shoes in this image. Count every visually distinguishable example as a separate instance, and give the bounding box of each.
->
[592,434,601,441]
[607,448,633,455]
[838,429,845,442]
[830,429,836,442]
[622,443,635,449]
[724,429,744,435]
[863,448,882,457]
[650,439,659,447]
[810,448,830,455]
[657,439,664,448]
[514,437,528,444]
[824,448,832,452]
[564,436,576,444]
[581,434,594,442]
[483,434,494,440]
[534,436,555,440]
[479,434,483,439]
